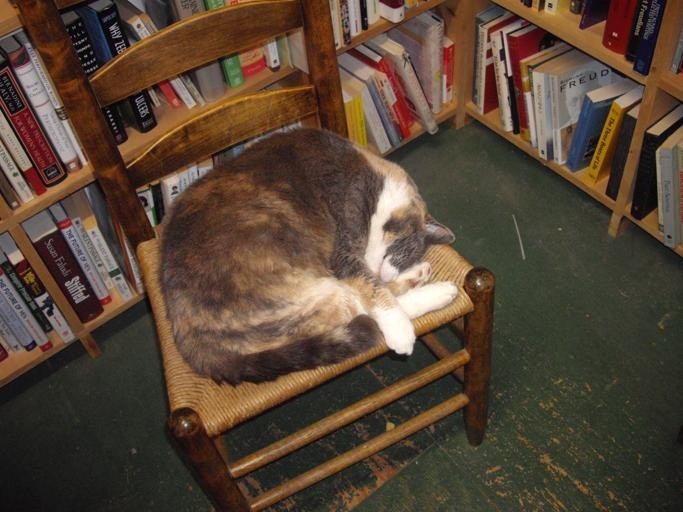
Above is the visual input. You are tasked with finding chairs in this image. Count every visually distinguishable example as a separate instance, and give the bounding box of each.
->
[15,3,496,512]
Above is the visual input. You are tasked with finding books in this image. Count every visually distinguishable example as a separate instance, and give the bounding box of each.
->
[1,183,144,361]
[521,1,668,76]
[631,104,683,250]
[328,0,454,154]
[135,118,301,228]
[1,30,88,210]
[671,31,683,73]
[472,6,643,201]
[60,0,279,142]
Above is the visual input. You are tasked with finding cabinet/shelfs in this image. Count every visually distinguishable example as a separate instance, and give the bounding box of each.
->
[466,4,682,261]
[0,2,467,391]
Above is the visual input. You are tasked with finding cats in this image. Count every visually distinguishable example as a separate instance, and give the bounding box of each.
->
[147,121,460,392]
